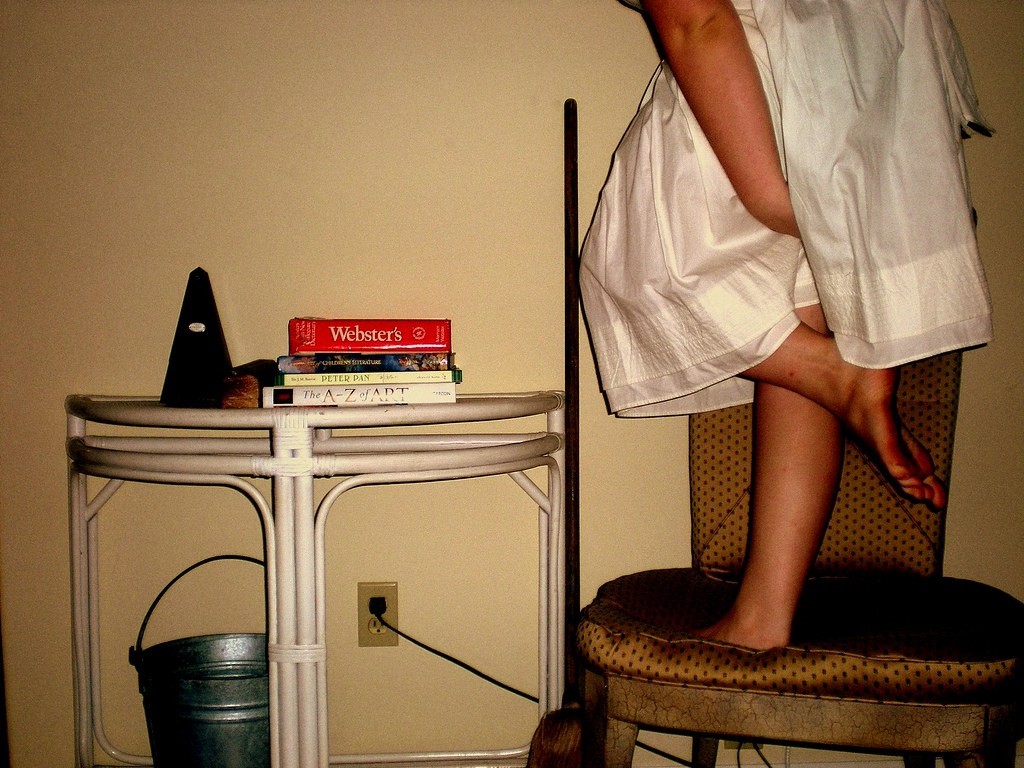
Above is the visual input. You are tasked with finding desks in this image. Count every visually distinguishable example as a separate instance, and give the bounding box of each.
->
[64,390,566,768]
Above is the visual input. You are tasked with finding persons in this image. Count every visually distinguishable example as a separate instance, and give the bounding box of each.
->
[577,0,991,653]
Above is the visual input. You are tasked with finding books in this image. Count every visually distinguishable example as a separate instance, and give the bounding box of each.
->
[262,318,464,409]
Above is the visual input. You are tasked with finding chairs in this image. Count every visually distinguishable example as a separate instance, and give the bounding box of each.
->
[573,352,1024,768]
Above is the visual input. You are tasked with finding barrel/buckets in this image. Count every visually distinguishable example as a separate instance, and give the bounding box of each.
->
[129,555,271,768]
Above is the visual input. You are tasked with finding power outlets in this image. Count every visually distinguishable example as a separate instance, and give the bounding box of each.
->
[357,582,399,648]
